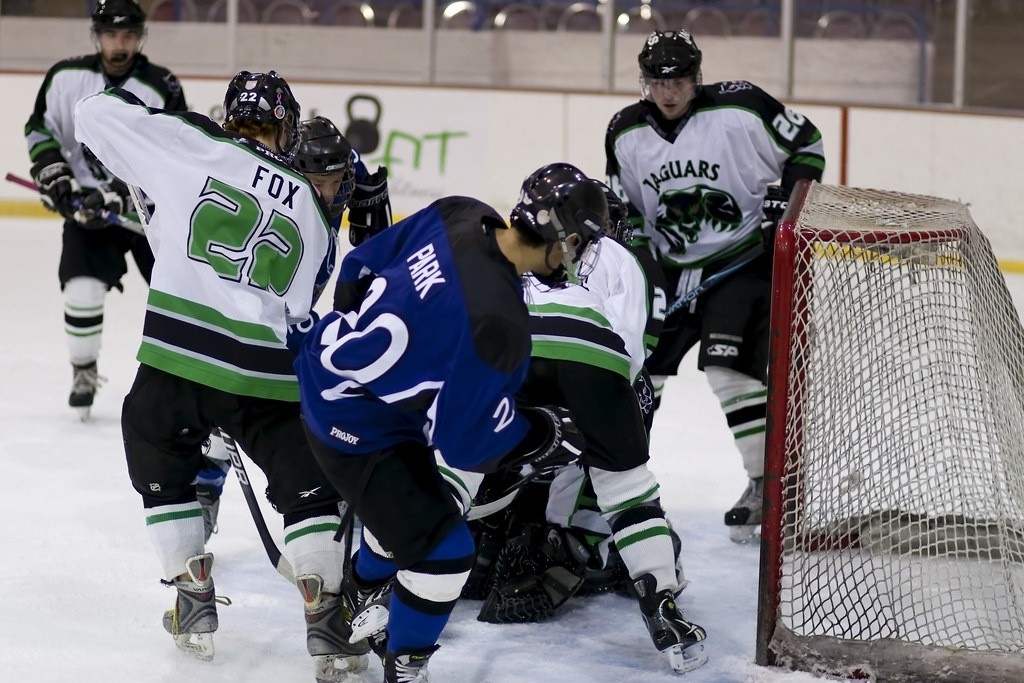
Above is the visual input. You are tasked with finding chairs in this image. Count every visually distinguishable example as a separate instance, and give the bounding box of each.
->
[151,0,923,40]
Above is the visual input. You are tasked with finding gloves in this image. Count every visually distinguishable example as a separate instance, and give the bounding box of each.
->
[526,406,585,478]
[74,178,134,228]
[759,193,790,260]
[348,164,395,248]
[30,153,83,212]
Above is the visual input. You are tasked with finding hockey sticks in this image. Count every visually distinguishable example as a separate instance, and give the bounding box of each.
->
[331,501,391,658]
[664,243,765,318]
[124,182,297,586]
[4,171,149,238]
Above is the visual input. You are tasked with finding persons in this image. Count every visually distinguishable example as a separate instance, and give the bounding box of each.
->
[295,162,609,683]
[192,116,393,543]
[521,178,708,675]
[70,69,370,683]
[601,29,826,544]
[25,0,188,422]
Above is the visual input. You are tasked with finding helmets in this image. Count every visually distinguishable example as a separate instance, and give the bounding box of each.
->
[637,28,703,80]
[218,68,302,123]
[511,161,609,293]
[289,115,356,173]
[591,175,633,249]
[91,0,149,33]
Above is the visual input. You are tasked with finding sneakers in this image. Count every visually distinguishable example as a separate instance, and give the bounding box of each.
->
[627,572,709,675]
[380,644,440,683]
[194,495,219,546]
[297,574,370,683]
[725,476,765,547]
[162,554,231,662]
[339,549,397,658]
[67,361,106,425]
